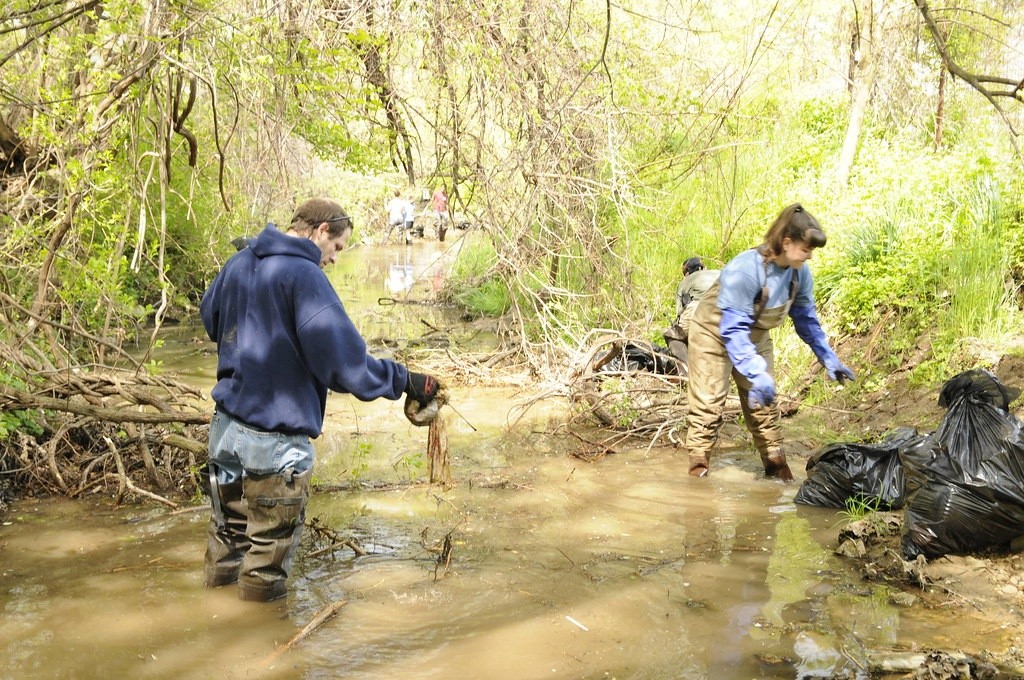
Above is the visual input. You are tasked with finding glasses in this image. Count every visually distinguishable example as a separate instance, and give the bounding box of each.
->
[313,216,354,228]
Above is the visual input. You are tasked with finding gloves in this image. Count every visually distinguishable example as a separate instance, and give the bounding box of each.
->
[788,303,856,385]
[719,309,777,410]
[404,370,440,409]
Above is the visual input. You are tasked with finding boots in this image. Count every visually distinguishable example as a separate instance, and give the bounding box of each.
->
[689,456,709,478]
[760,450,793,480]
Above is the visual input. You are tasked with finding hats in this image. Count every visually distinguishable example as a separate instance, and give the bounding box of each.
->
[682,258,704,276]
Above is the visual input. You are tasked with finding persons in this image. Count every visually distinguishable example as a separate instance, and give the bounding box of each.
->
[432,184,451,241]
[687,203,855,483]
[382,190,415,244]
[663,257,733,392]
[200,199,450,601]
